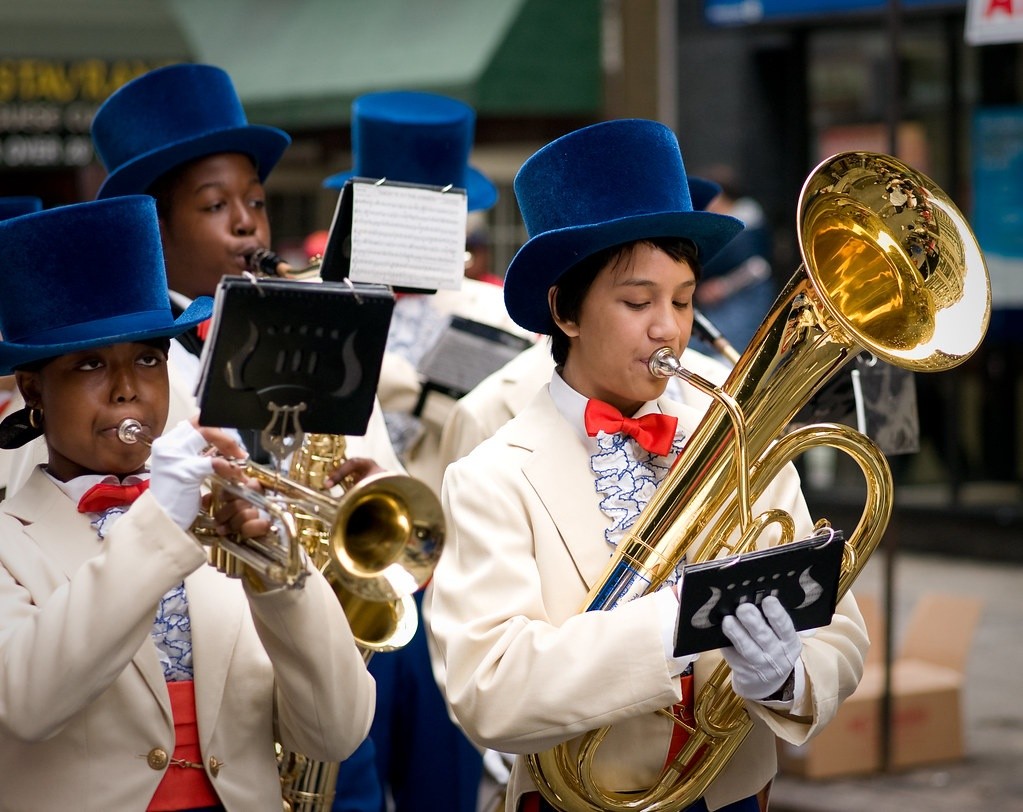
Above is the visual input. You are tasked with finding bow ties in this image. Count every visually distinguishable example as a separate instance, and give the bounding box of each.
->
[584,399,677,457]
[78,479,148,512]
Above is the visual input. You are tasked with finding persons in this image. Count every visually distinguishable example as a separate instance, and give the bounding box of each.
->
[0,196,377,812]
[88,63,781,812]
[421,118,870,812]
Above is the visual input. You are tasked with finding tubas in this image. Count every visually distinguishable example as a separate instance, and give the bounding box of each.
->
[524,148,994,812]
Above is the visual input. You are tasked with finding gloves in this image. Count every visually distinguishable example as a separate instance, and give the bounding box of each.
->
[720,593,801,700]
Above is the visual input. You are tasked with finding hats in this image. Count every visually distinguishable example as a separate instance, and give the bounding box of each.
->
[686,174,721,211]
[0,195,215,376]
[322,91,499,212]
[503,119,746,334]
[93,63,290,202]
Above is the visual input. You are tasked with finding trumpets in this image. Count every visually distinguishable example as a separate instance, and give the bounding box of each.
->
[116,418,449,602]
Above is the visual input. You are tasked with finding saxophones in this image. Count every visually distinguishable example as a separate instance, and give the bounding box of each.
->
[202,245,420,810]
